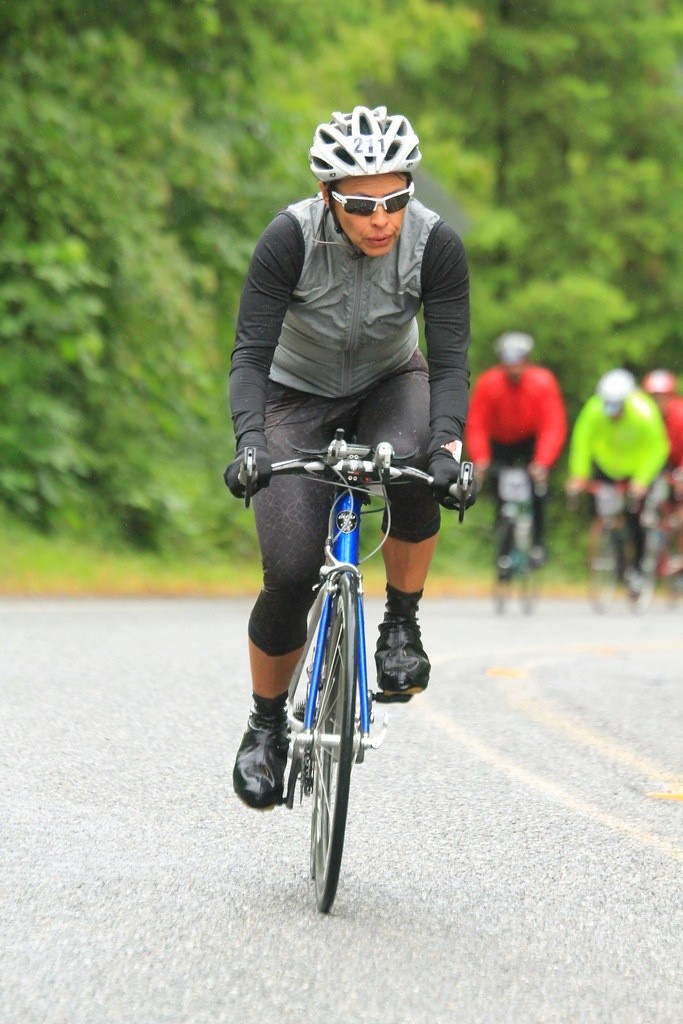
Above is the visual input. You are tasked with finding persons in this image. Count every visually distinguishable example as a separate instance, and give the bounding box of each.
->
[464,330,682,608]
[223,105,475,812]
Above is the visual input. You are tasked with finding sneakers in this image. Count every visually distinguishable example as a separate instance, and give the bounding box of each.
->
[374,617,430,694]
[233,721,289,812]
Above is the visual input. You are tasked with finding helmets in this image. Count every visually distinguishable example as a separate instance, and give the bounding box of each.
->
[309,106,422,183]
[495,333,533,359]
[598,369,635,401]
[642,369,676,393]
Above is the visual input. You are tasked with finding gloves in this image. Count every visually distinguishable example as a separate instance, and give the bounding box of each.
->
[222,445,273,498]
[427,448,477,511]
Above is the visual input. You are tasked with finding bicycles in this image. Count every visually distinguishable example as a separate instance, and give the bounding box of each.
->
[474,456,683,617]
[238,427,478,918]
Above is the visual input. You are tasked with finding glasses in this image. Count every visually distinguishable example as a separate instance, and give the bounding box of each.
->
[332,180,415,217]
